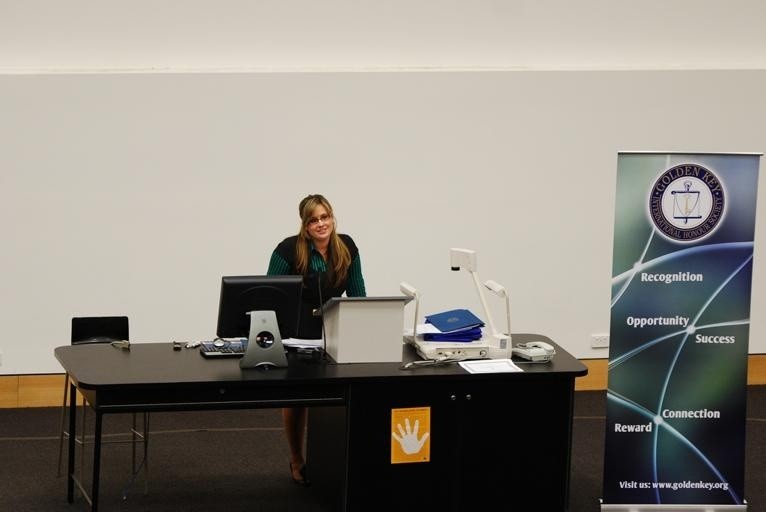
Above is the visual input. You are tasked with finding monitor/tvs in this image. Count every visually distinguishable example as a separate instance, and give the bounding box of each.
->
[217,275,305,369]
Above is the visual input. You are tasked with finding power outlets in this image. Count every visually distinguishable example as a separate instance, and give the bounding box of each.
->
[591,335,610,348]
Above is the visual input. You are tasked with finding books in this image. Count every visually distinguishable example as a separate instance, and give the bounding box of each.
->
[418,308,486,342]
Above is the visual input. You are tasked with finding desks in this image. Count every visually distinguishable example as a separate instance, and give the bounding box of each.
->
[53,331,589,512]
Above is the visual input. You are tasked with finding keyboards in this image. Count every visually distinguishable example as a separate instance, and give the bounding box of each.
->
[200,340,288,356]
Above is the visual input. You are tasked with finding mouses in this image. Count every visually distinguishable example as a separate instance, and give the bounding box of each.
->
[213,338,225,347]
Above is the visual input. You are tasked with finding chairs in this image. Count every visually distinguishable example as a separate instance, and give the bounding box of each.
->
[56,316,152,495]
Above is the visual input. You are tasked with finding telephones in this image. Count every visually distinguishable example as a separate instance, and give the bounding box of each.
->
[512,341,556,362]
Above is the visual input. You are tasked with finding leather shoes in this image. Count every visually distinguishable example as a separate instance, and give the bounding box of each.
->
[290,461,310,486]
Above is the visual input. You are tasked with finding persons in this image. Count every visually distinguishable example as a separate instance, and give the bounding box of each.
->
[266,194,367,482]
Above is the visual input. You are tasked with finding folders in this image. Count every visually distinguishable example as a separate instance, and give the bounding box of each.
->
[424,309,485,342]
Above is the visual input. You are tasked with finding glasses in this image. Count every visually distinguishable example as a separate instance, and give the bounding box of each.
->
[308,214,331,224]
